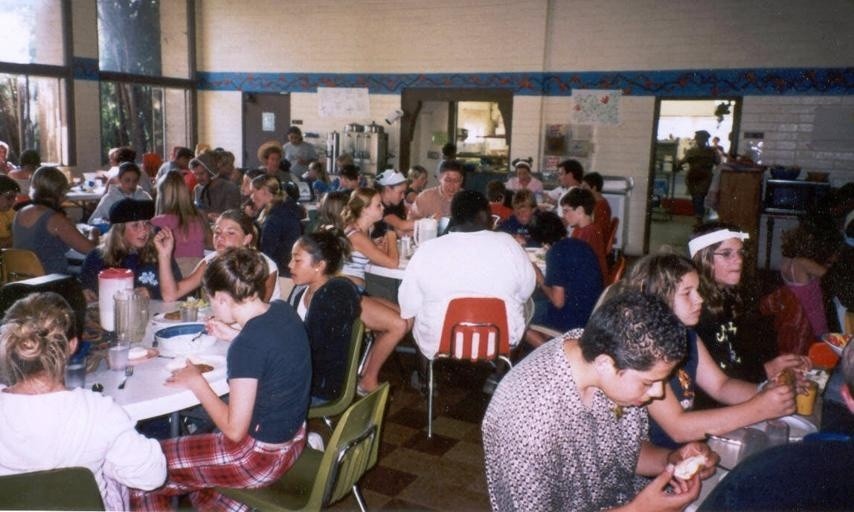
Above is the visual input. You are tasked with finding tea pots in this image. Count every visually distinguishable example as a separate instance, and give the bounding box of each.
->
[413,218,438,248]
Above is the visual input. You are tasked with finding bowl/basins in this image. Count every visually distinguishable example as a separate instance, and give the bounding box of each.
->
[154,324,218,353]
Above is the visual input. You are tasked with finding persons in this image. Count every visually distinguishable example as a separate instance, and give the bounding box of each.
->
[482,292,719,512]
[674,131,733,226]
[93,127,611,394]
[698,338,854,511]
[284,229,412,407]
[627,253,795,447]
[129,247,311,512]
[768,215,853,353]
[0,293,168,511]
[688,224,813,382]
[0,136,95,273]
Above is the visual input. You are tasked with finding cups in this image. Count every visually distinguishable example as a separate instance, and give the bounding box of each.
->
[112,288,149,349]
[795,380,818,418]
[179,305,198,322]
[107,341,129,370]
[301,171,310,179]
[64,359,87,390]
[98,268,134,331]
[397,240,409,257]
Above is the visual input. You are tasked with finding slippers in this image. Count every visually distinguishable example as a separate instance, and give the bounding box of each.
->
[357,385,394,401]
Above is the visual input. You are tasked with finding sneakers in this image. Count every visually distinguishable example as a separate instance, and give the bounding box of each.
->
[307,431,325,452]
[412,370,438,397]
[482,372,502,395]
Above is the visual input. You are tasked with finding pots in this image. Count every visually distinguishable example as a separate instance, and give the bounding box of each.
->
[364,120,384,133]
[344,123,364,132]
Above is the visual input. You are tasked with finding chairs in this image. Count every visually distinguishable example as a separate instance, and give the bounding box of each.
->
[306,318,375,510]
[1,467,105,510]
[528,256,625,338]
[217,380,391,511]
[1,248,45,284]
[605,217,619,263]
[419,297,513,439]
[278,276,294,301]
[176,257,202,280]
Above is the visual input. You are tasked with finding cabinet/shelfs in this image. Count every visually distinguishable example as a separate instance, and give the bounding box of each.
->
[718,170,765,275]
[757,215,800,273]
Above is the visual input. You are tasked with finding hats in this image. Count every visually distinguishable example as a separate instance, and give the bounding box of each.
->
[188,152,220,205]
[110,198,156,223]
[377,169,409,186]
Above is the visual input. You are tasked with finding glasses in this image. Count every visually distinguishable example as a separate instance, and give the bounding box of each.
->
[712,250,748,260]
[128,222,154,232]
[1,188,15,200]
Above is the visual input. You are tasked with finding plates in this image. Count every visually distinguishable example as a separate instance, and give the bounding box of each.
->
[151,311,212,328]
[821,332,850,357]
[744,414,819,442]
[159,354,228,381]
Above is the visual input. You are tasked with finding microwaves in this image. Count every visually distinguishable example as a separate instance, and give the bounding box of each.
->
[763,179,831,215]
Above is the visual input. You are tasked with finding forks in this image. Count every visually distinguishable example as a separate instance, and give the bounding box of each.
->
[117,364,134,389]
